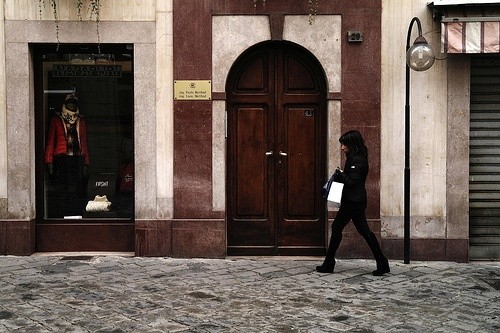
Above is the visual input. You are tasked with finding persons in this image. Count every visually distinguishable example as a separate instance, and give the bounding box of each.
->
[46,99,90,217]
[316,130,390,276]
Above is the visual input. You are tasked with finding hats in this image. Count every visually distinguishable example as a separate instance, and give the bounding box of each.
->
[65,93,78,101]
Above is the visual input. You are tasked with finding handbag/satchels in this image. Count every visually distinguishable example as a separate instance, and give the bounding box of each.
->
[323,166,344,204]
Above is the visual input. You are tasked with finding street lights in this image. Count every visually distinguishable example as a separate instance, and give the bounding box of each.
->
[403,16,436,263]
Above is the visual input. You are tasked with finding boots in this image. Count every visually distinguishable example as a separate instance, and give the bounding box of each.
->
[372,258,390,276]
[316,257,336,273]
